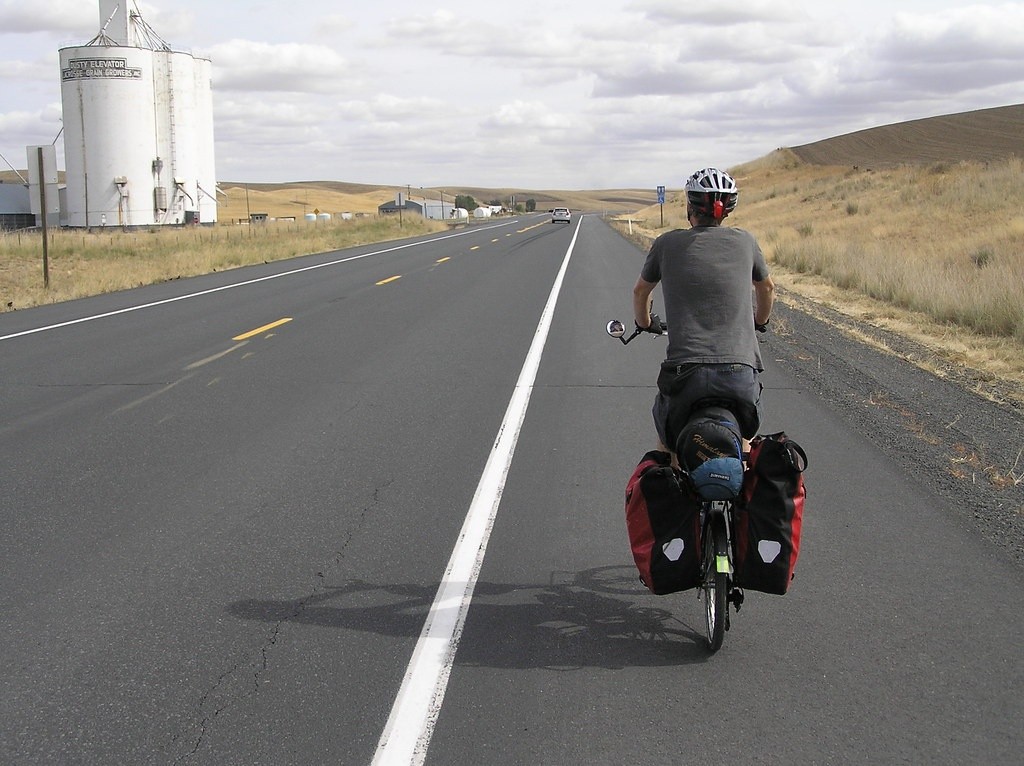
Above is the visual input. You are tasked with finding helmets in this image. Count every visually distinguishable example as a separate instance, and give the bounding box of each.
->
[684,168,738,219]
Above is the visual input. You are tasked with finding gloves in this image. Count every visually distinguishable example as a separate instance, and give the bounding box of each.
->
[754,313,770,331]
[635,314,662,334]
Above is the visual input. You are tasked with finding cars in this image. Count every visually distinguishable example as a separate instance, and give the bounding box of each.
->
[551,207,571,224]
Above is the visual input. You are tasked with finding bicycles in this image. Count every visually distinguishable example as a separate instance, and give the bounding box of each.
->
[606,299,770,651]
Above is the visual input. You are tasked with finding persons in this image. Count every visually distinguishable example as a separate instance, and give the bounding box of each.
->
[632,167,774,471]
[609,321,624,337]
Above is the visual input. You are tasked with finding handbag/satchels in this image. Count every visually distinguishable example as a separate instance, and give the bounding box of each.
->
[737,431,808,595]
[625,450,701,596]
